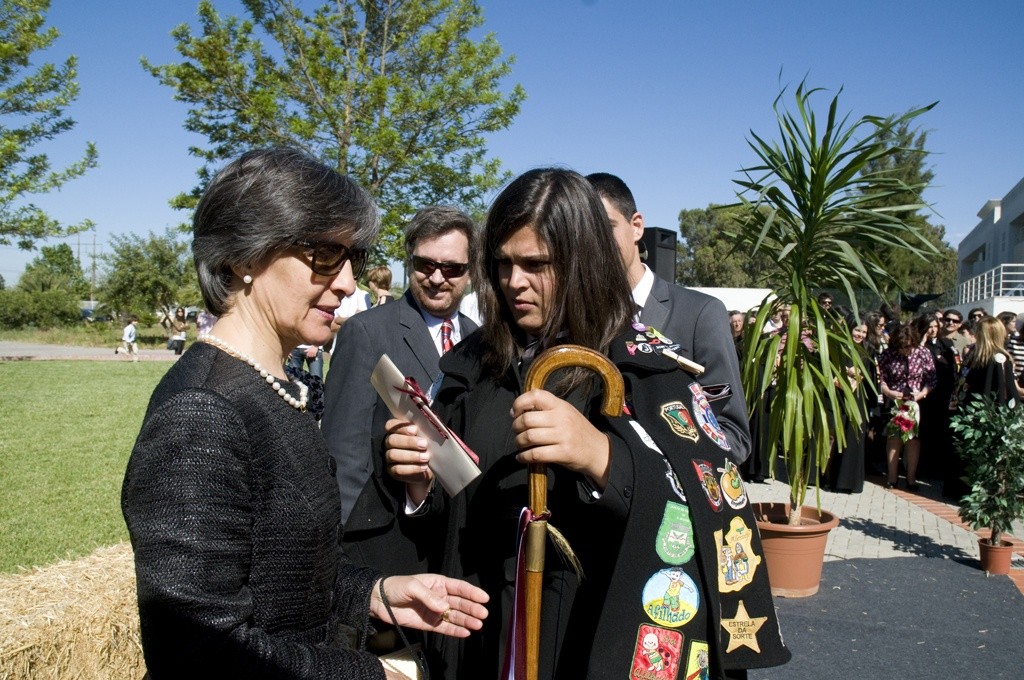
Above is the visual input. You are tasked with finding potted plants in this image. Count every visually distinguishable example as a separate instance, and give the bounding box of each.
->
[950,393,1024,575]
[740,63,941,598]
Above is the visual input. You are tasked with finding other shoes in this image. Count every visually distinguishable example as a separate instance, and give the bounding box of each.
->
[906,479,917,492]
[884,478,898,489]
[115,346,120,355]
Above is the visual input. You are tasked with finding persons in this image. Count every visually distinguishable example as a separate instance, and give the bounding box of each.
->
[115,315,139,361]
[586,172,751,466]
[727,293,1024,495]
[343,167,795,680]
[120,145,491,680]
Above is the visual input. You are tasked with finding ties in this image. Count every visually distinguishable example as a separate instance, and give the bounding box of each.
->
[629,301,641,316]
[438,318,455,355]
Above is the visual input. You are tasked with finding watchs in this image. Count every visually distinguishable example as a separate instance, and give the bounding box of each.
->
[921,392,926,398]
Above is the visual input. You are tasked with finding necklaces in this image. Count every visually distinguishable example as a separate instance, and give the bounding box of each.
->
[196,334,309,414]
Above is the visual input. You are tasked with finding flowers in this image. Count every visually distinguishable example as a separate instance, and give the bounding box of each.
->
[886,394,920,443]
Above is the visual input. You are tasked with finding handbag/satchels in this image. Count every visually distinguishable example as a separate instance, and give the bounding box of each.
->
[166,340,178,350]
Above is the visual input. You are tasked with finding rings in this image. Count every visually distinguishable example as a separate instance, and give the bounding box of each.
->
[443,609,451,622]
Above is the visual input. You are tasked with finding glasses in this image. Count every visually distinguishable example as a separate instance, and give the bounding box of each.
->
[943,317,961,325]
[877,323,887,328]
[285,237,370,280]
[971,314,983,319]
[821,300,832,305]
[409,255,469,278]
